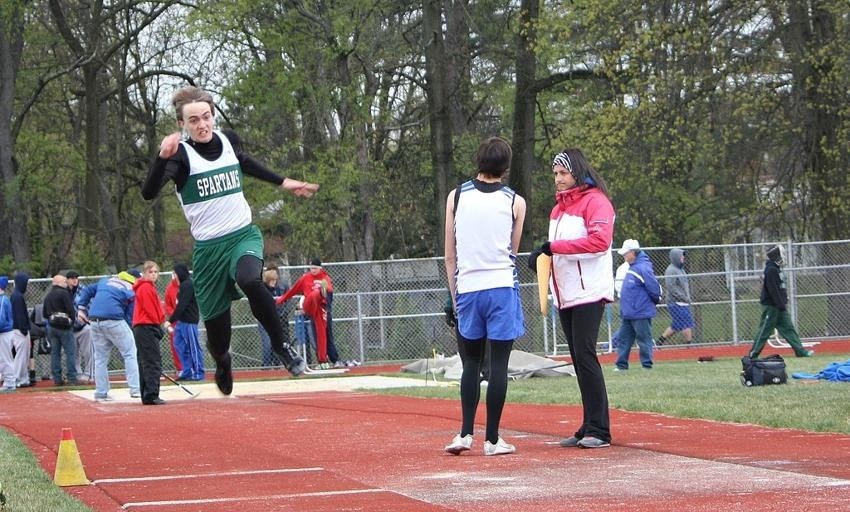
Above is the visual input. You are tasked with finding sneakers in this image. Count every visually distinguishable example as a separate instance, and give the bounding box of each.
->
[575,435,612,450]
[483,435,516,456]
[214,352,233,395]
[313,359,362,370]
[277,341,308,377]
[444,432,474,455]
[559,435,579,448]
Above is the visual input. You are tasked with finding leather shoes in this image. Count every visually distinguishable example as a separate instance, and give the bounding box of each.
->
[142,397,165,405]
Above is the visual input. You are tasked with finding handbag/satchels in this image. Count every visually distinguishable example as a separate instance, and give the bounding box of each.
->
[738,355,788,387]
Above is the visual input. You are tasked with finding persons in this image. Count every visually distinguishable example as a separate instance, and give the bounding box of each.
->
[600,260,630,355]
[141,86,320,390]
[444,137,527,457]
[749,245,814,359]
[42,275,89,385]
[652,249,696,352]
[527,148,616,449]
[613,239,666,372]
[165,263,205,381]
[66,270,95,381]
[78,269,140,402]
[0,276,17,391]
[258,264,292,370]
[277,261,340,369]
[133,261,165,405]
[10,273,33,387]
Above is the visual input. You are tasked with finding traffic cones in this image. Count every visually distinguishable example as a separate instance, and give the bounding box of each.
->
[51,426,93,486]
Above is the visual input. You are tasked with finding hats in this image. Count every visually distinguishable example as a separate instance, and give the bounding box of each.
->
[66,271,80,278]
[309,257,321,267]
[616,238,642,257]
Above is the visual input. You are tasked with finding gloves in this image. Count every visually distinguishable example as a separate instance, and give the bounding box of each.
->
[528,247,543,270]
